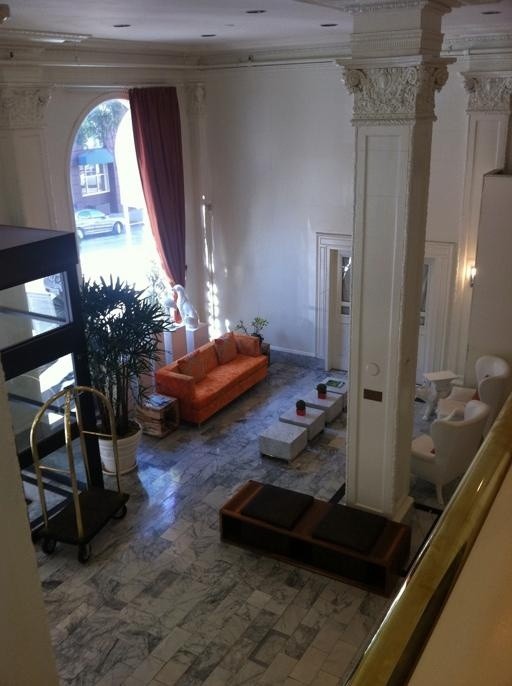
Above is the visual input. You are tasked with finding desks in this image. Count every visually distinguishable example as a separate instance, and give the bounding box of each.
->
[261,343,270,366]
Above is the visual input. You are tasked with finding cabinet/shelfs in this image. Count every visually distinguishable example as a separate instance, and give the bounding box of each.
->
[0,225,104,546]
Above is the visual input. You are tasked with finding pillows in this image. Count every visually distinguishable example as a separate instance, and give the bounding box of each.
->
[214,331,237,365]
[177,350,206,384]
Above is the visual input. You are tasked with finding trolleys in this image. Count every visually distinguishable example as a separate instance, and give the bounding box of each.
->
[33,386,130,564]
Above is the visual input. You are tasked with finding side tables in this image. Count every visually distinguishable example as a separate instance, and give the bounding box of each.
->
[133,392,180,438]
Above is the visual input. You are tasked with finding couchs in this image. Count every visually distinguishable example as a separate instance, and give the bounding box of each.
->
[154,331,267,430]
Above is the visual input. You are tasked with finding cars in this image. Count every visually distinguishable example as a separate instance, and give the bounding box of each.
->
[80,164,97,186]
[74,207,126,240]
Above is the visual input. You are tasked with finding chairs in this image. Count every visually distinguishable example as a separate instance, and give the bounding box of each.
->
[410,355,512,505]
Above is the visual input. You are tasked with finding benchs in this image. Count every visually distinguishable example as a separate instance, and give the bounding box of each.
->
[219,480,411,598]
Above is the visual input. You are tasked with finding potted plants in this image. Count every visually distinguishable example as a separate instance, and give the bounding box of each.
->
[54,275,177,472]
[234,312,269,346]
